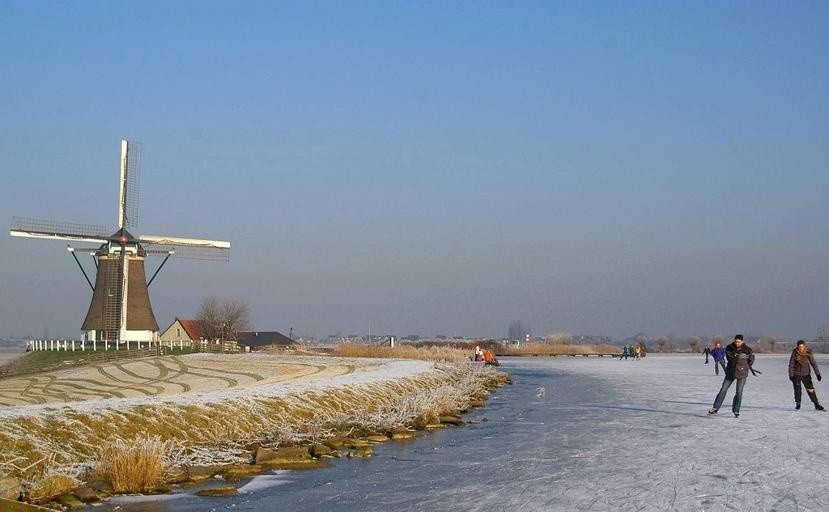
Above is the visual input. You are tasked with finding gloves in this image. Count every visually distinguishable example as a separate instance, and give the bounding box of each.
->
[789,376,795,382]
[817,373,821,381]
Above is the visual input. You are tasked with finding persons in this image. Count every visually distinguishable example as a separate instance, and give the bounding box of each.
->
[708,334,755,416]
[702,345,710,364]
[788,339,824,411]
[474,345,500,367]
[619,344,640,361]
[710,342,726,376]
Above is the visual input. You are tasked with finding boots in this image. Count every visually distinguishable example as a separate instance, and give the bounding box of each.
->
[795,403,800,409]
[815,402,824,410]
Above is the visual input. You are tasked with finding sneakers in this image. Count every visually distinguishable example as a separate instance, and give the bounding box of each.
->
[709,408,717,413]
[734,411,739,415]
